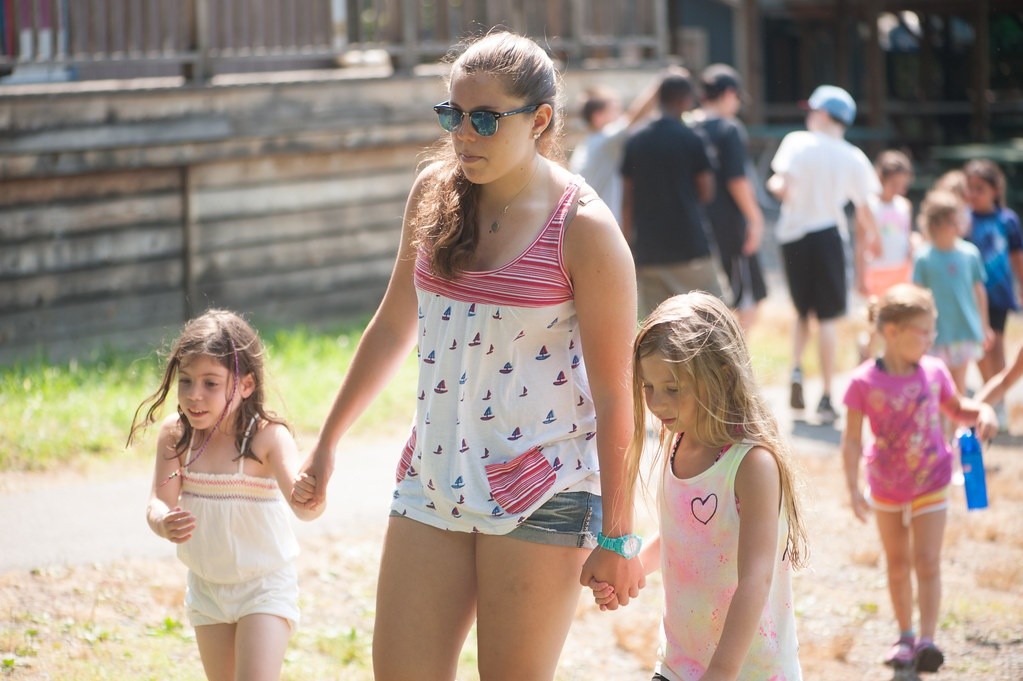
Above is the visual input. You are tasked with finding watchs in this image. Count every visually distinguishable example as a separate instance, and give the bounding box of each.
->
[596,533,644,560]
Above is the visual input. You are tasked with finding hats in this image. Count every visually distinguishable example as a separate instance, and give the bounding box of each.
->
[799,84,857,127]
[704,63,755,106]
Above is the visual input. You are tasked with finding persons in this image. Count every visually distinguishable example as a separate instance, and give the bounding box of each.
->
[590,291,803,681]
[578,62,767,343]
[847,282,1000,673]
[291,31,646,681]
[970,345,1023,407]
[146,309,325,681]
[859,150,1023,437]
[769,85,870,427]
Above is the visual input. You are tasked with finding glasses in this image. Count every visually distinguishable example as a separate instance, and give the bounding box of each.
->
[434,102,536,137]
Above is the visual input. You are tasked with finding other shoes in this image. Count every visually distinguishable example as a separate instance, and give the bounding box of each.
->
[919,642,943,671]
[791,383,803,409]
[885,636,916,666]
[817,396,838,418]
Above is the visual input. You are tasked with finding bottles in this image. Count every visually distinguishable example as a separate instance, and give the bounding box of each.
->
[958,426,988,509]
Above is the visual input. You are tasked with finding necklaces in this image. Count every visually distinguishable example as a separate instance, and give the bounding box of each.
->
[473,163,539,235]
